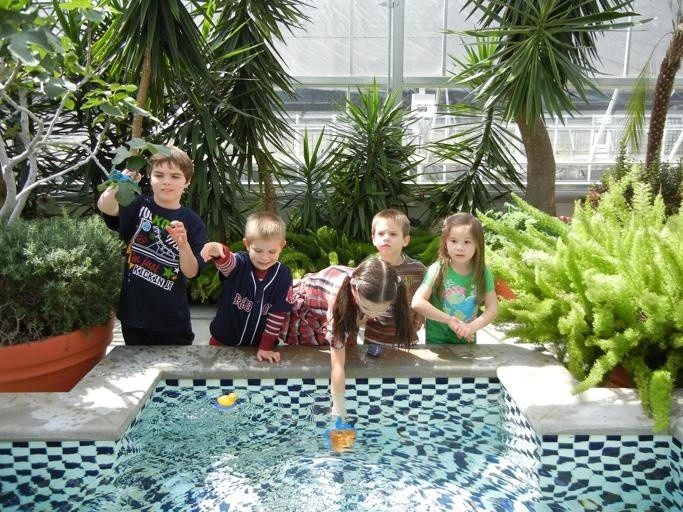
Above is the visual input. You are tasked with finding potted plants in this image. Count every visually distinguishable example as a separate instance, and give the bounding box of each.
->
[488,159,682,434]
[0,216,127,396]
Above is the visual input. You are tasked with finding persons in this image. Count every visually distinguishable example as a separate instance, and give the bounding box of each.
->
[411,213,499,345]
[200,212,294,365]
[363,209,431,347]
[97,145,207,345]
[287,257,407,426]
